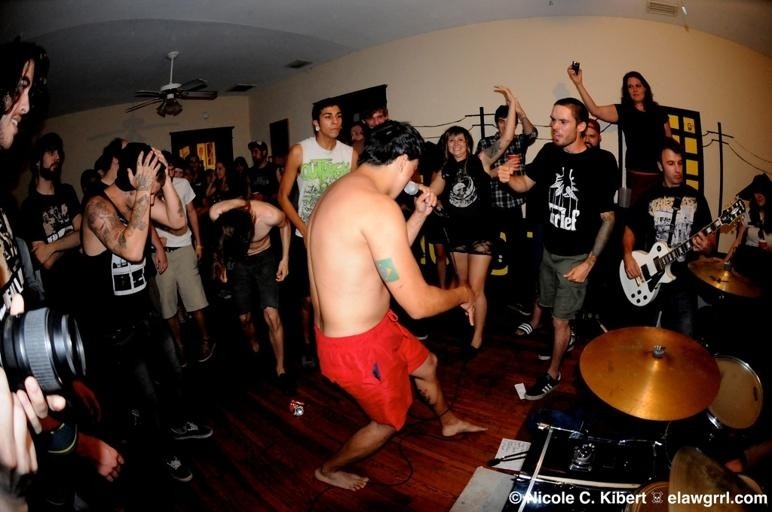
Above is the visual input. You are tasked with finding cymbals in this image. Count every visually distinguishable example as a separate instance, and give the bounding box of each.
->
[579,327,719,420]
[691,262,753,296]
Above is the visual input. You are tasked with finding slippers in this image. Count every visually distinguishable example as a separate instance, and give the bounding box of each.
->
[514,321,545,339]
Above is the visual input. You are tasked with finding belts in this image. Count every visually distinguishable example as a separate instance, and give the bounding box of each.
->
[151,247,178,252]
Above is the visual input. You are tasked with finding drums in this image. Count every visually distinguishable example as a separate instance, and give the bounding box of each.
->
[708,354,763,428]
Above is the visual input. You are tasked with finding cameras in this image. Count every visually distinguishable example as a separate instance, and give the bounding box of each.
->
[0,302,93,404]
[572,60,582,75]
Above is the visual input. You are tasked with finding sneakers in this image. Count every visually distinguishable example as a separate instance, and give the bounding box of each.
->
[197,334,217,363]
[506,303,532,316]
[166,455,193,483]
[170,419,214,441]
[525,371,561,401]
[176,344,189,368]
[539,335,575,361]
[407,328,428,339]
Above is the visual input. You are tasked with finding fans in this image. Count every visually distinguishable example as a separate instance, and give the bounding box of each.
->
[126,78,218,113]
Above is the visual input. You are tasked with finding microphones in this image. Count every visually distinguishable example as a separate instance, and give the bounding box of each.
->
[404,182,443,218]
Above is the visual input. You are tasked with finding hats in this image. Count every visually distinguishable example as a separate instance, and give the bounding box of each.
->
[115,142,165,190]
[249,140,266,150]
[736,173,772,200]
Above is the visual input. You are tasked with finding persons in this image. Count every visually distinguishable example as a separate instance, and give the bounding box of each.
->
[348,120,368,145]
[566,65,673,216]
[561,118,622,356]
[78,153,105,194]
[170,140,285,206]
[413,84,517,361]
[473,84,538,316]
[305,117,488,494]
[719,172,772,285]
[0,32,126,511]
[207,194,293,398]
[80,141,214,484]
[361,102,389,133]
[78,133,129,250]
[146,148,217,367]
[496,96,622,401]
[1,361,68,512]
[515,270,544,338]
[622,136,718,338]
[18,130,148,444]
[275,99,361,369]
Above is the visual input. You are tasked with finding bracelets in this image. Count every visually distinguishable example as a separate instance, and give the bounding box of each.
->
[195,242,203,249]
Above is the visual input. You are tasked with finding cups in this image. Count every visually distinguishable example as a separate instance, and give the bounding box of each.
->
[508,154,519,170]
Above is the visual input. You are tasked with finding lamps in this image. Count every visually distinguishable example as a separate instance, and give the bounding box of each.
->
[156,50,183,117]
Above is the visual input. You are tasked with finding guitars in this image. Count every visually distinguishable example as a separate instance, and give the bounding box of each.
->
[620,199,746,307]
